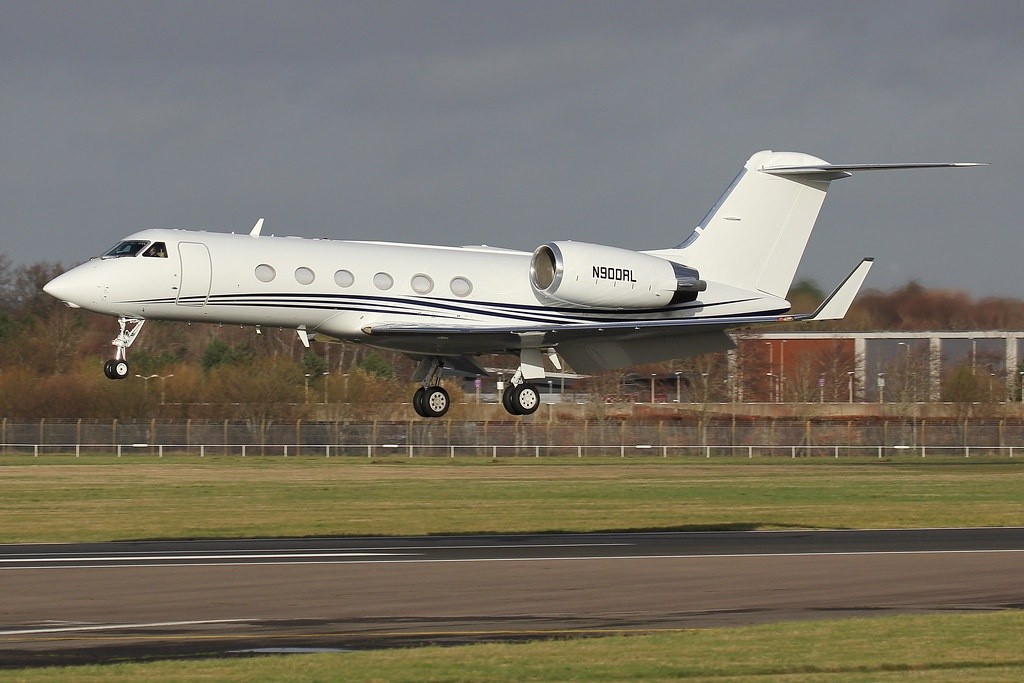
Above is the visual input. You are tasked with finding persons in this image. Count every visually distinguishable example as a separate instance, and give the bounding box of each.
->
[148,245,160,257]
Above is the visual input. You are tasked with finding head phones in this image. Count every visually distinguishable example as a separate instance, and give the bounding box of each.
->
[153,248,156,251]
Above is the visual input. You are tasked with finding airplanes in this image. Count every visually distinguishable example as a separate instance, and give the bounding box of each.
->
[40,150,992,417]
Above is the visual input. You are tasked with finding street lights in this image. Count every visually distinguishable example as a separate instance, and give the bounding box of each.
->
[473,372,1024,403]
[780,340,787,400]
[899,342,910,378]
[765,342,774,399]
[304,372,351,403]
[134,374,173,406]
